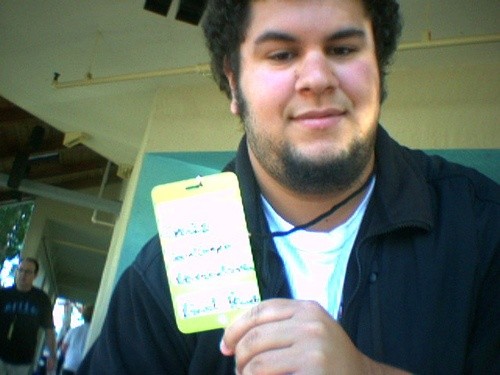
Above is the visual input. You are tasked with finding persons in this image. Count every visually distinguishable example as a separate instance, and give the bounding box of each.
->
[54,325,72,375]
[60,305,93,375]
[75,0,500,375]
[0,256,58,375]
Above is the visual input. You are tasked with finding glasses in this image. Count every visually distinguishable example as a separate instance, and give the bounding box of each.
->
[17,268,34,275]
